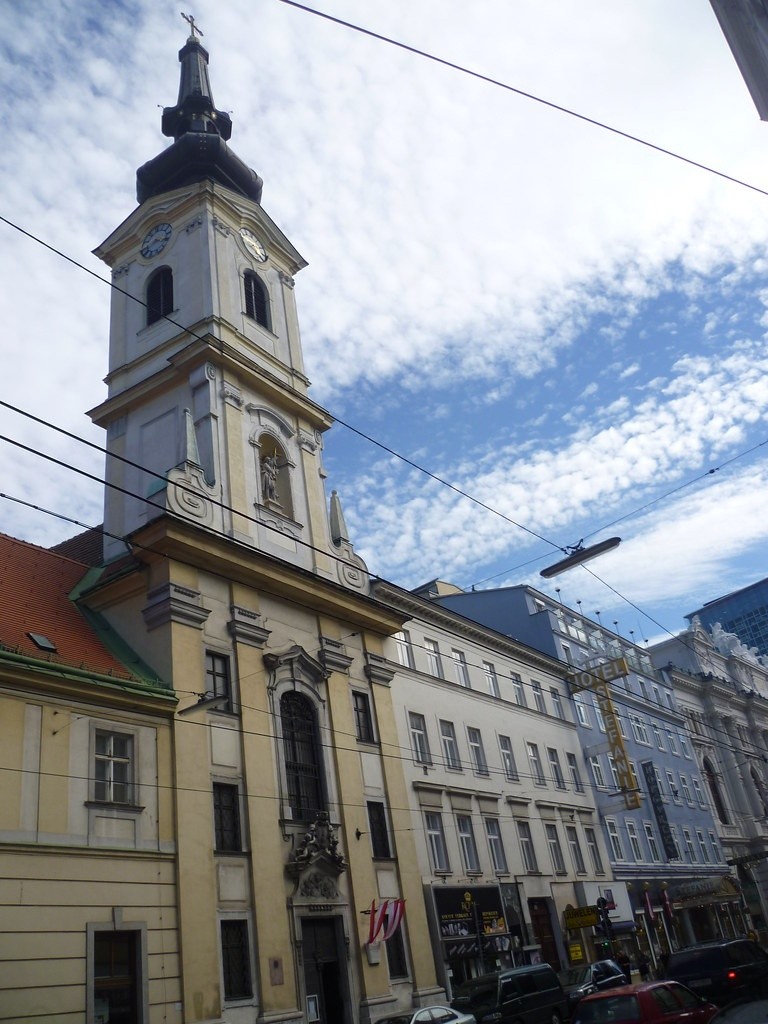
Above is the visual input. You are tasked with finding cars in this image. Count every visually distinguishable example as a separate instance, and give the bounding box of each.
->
[706,993,768,1024]
[372,1005,478,1024]
[573,979,720,1024]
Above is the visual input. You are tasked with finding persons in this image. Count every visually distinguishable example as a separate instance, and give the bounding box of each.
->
[617,950,633,983]
[635,950,654,981]
[690,613,768,667]
[741,929,758,942]
[261,455,279,501]
[293,811,346,864]
[660,950,670,967]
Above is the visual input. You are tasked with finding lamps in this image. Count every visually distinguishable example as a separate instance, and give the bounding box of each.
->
[541,536,622,578]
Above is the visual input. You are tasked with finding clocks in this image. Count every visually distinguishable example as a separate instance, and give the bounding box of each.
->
[238,228,267,262]
[141,223,172,259]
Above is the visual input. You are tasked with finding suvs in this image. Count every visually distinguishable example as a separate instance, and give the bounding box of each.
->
[449,963,572,1024]
[666,936,768,1008]
[555,958,629,1015]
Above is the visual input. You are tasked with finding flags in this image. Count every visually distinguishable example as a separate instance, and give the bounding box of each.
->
[368,901,375,943]
[383,901,405,939]
[382,899,398,937]
[365,901,388,944]
